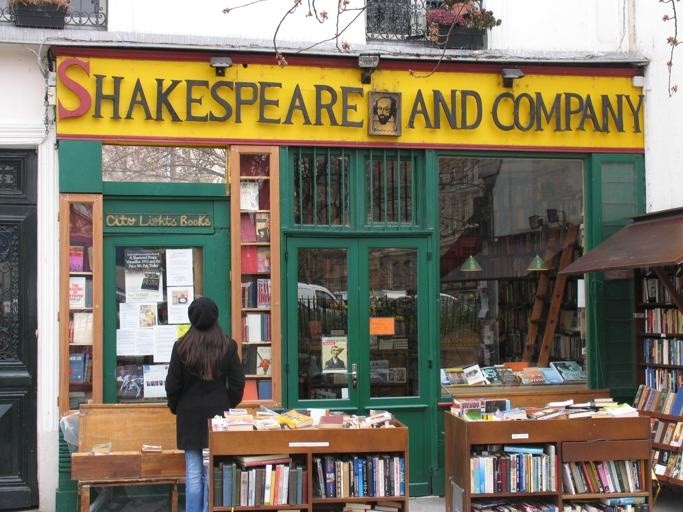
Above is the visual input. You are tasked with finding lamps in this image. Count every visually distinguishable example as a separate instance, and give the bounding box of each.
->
[356,53,381,86]
[210,55,233,77]
[502,65,525,89]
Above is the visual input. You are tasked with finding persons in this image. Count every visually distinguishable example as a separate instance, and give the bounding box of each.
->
[373,96,396,132]
[164,298,245,511]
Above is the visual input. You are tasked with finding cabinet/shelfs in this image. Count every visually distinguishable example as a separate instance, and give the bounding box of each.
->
[483,226,582,363]
[633,272,683,499]
[58,190,104,411]
[231,145,282,404]
[205,409,409,512]
[442,408,650,512]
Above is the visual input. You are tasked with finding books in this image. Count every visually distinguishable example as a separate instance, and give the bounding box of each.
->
[436,156,683,512]
[68,200,93,409]
[210,153,421,512]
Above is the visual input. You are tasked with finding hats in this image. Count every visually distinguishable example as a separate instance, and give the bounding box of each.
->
[188,297,219,332]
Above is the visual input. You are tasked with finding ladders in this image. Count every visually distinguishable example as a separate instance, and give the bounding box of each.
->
[520,197,582,367]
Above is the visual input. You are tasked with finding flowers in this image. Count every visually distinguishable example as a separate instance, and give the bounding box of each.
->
[426,1,503,28]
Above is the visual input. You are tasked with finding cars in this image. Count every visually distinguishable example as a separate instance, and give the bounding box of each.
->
[371,290,471,314]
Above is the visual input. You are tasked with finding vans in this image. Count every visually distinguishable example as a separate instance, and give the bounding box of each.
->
[296,282,345,316]
